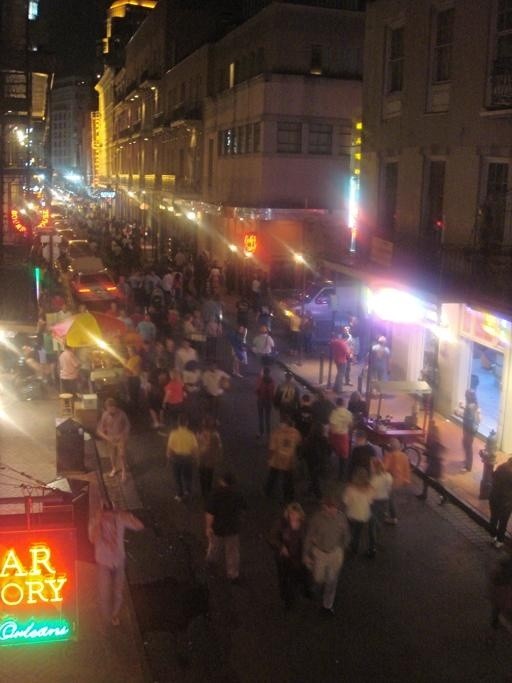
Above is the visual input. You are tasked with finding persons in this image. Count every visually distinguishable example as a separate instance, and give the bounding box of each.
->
[489,453,511,548]
[457,384,482,473]
[479,539,511,630]
[26,194,453,632]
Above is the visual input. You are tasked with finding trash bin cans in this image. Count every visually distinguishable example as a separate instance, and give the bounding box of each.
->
[56,416,86,475]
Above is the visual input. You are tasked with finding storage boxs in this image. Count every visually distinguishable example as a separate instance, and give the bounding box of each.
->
[82,393,98,409]
[74,400,97,426]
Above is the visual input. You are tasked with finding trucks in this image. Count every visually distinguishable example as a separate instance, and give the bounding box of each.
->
[278,279,358,323]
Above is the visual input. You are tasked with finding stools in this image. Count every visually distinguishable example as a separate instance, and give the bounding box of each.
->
[59,393,73,418]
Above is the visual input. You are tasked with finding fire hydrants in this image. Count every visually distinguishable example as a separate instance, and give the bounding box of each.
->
[478,428,499,500]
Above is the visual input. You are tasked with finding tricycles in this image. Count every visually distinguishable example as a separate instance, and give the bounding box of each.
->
[358,378,432,474]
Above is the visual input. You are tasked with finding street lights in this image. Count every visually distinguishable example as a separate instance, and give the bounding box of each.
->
[363,289,376,407]
[320,288,340,392]
[141,188,149,257]
[293,250,305,366]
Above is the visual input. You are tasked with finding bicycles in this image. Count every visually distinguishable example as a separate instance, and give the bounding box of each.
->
[235,288,272,313]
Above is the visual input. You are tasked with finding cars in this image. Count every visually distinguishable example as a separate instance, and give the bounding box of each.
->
[49,213,119,304]
